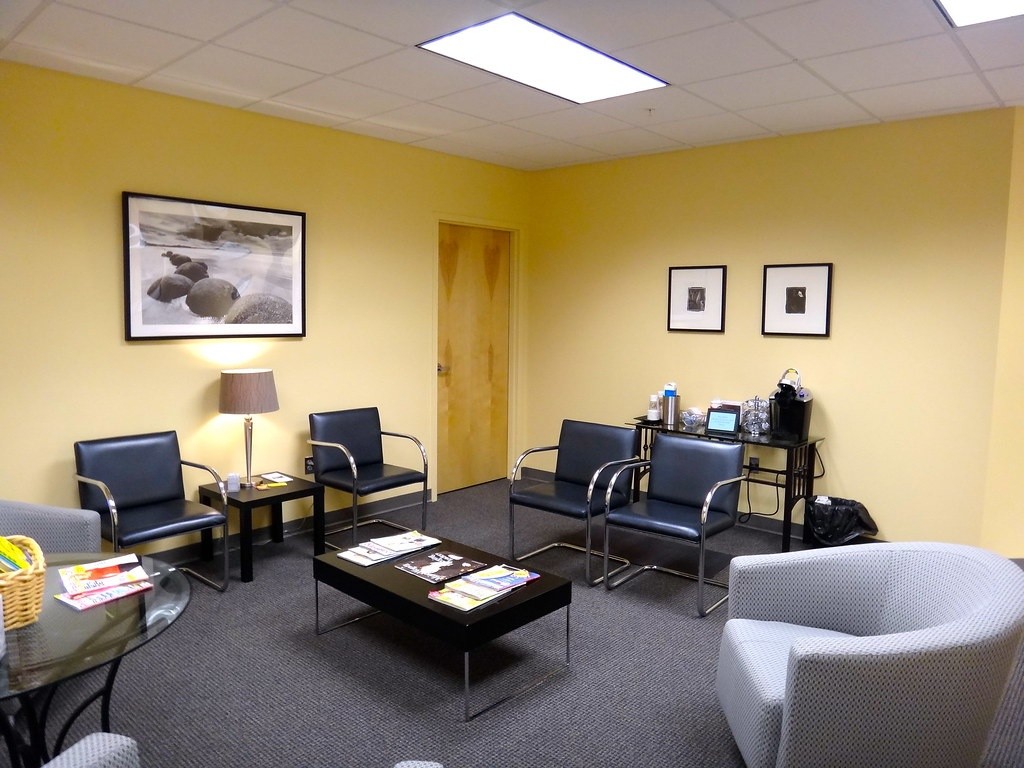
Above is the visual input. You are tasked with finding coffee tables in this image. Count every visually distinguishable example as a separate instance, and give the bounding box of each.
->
[312,531,574,721]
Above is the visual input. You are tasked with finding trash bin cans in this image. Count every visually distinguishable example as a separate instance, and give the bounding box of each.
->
[804,495,862,550]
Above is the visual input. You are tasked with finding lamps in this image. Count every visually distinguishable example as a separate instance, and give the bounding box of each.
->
[218,367,278,486]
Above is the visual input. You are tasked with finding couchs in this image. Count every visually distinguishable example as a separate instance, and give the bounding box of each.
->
[0,499,101,553]
[41,732,445,768]
[716,541,1023,768]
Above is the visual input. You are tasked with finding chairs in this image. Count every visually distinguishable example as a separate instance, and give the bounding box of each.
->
[603,435,751,617]
[73,430,231,592]
[305,408,428,554]
[508,418,639,586]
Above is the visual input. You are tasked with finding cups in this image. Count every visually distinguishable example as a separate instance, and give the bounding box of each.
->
[646,390,680,425]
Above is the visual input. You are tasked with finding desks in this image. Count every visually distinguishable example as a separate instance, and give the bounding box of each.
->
[199,471,325,582]
[626,413,826,552]
[0,550,192,768]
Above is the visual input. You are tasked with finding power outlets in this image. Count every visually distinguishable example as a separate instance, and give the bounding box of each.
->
[304,456,314,474]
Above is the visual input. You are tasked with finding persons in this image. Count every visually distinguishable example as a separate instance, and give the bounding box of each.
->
[74,562,141,580]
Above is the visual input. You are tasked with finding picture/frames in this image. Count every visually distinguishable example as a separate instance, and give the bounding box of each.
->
[762,263,832,337]
[121,191,307,341]
[668,264,727,332]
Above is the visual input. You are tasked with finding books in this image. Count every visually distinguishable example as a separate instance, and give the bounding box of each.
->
[336,530,540,612]
[53,553,154,612]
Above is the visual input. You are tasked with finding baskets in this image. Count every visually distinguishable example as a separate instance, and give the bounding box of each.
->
[0,534,46,632]
